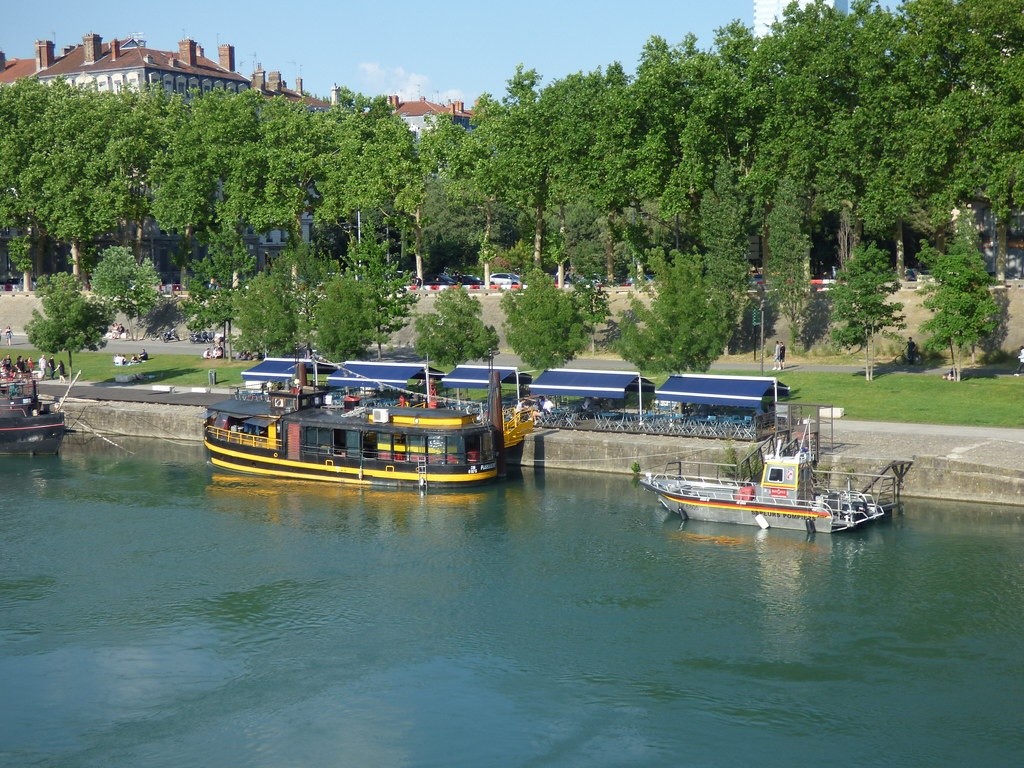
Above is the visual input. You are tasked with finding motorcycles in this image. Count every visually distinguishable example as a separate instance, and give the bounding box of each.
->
[162,326,180,343]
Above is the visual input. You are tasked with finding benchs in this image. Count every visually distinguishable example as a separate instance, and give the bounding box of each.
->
[152,385,175,392]
[820,408,844,418]
[104,332,127,339]
[20,371,43,378]
[246,381,267,389]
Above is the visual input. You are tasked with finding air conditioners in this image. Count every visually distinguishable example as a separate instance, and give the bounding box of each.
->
[373,408,390,423]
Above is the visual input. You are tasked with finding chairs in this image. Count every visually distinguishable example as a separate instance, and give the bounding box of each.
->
[732,415,741,421]
[707,416,716,420]
[744,416,752,429]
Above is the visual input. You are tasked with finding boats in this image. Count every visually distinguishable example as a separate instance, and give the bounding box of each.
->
[638,413,895,534]
[0,367,83,456]
[196,337,506,489]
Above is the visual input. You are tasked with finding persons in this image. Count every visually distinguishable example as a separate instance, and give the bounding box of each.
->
[113,349,148,365]
[110,323,124,339]
[0,329,2,341]
[904,337,916,365]
[772,341,785,370]
[1014,346,1024,377]
[5,326,13,346]
[0,354,66,384]
[203,347,253,360]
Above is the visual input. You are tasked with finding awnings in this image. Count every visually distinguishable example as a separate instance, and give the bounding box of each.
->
[326,361,447,388]
[442,365,532,390]
[529,368,653,398]
[654,374,790,407]
[241,358,339,382]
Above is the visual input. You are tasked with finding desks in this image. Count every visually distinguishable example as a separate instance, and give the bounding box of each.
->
[360,397,753,439]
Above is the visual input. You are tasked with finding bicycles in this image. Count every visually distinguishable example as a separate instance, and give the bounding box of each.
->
[895,349,923,367]
[150,327,165,341]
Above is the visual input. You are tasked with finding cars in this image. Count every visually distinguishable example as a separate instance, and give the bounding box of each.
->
[452,274,484,286]
[422,273,458,286]
[489,272,521,286]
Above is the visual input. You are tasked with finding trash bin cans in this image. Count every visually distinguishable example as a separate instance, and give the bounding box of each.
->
[208,369,216,385]
